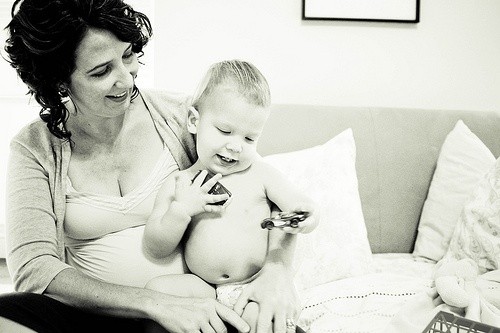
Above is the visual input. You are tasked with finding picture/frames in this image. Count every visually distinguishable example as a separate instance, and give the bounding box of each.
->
[302,0,420,23]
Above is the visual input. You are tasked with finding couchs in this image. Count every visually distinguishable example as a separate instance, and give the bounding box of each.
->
[1,102,500,332]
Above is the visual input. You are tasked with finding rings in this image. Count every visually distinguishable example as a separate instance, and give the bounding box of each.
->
[287,321,295,330]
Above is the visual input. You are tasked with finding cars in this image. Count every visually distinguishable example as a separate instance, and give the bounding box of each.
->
[261,209,309,231]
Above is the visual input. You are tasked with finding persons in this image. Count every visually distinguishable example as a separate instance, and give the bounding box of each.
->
[144,62,320,333]
[0,0,304,333]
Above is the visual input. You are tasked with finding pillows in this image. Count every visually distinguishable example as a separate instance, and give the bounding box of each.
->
[264,130,377,261]
[413,120,496,262]
